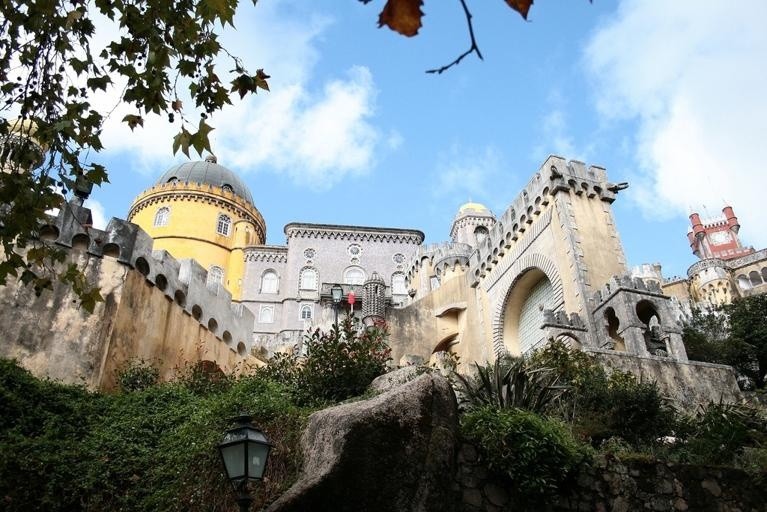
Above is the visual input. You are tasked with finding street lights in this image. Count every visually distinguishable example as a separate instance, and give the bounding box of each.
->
[218,413,270,511]
[329,282,343,351]
